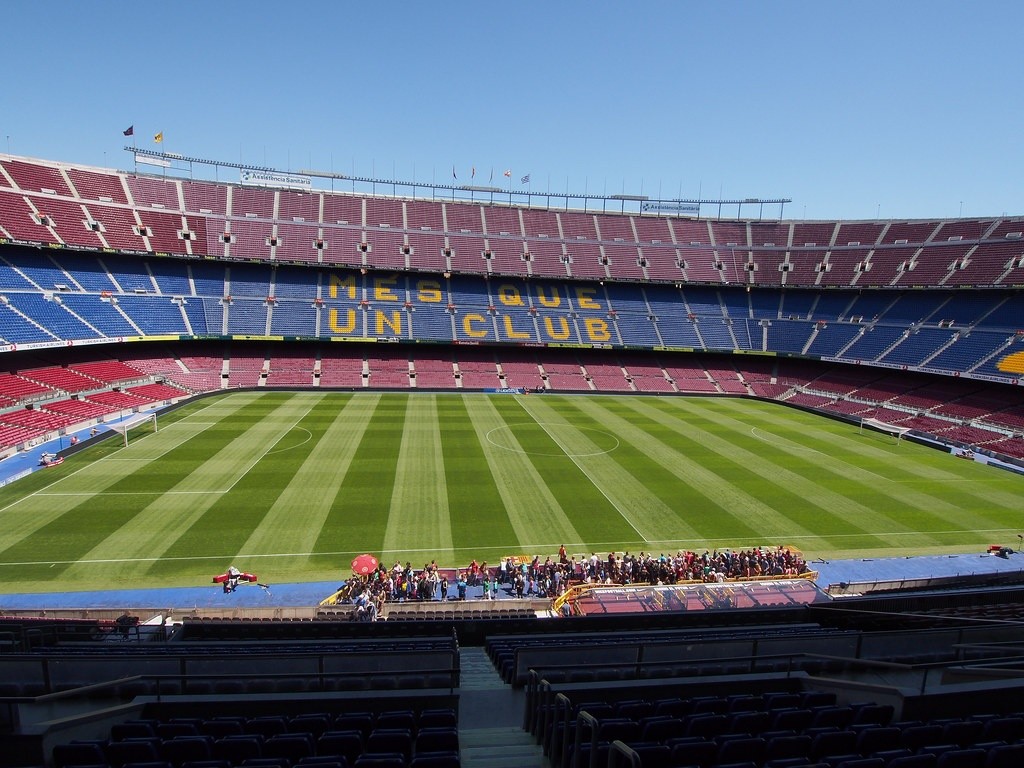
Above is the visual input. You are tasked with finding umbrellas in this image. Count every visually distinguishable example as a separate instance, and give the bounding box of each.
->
[351,554,378,580]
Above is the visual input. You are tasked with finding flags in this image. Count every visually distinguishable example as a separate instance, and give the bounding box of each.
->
[154,133,162,142]
[123,126,133,136]
[504,172,510,177]
[454,172,457,179]
[521,176,529,184]
[472,170,475,178]
[489,172,492,183]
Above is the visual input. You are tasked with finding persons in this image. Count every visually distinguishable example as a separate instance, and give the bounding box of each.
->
[536,385,540,392]
[90,428,98,437]
[70,436,76,446]
[335,545,807,620]
[541,385,545,393]
